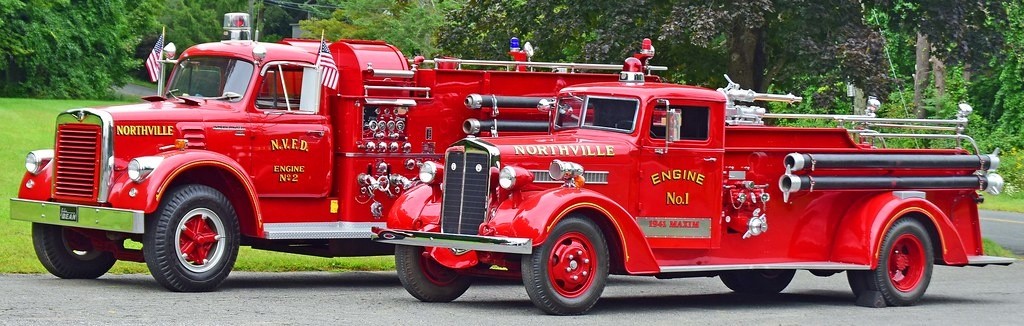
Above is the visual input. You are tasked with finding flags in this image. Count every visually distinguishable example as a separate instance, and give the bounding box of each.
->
[145,29,163,82]
[314,37,339,89]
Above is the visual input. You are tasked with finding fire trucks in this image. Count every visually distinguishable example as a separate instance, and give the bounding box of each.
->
[370,54,1015,317]
[10,12,672,294]
